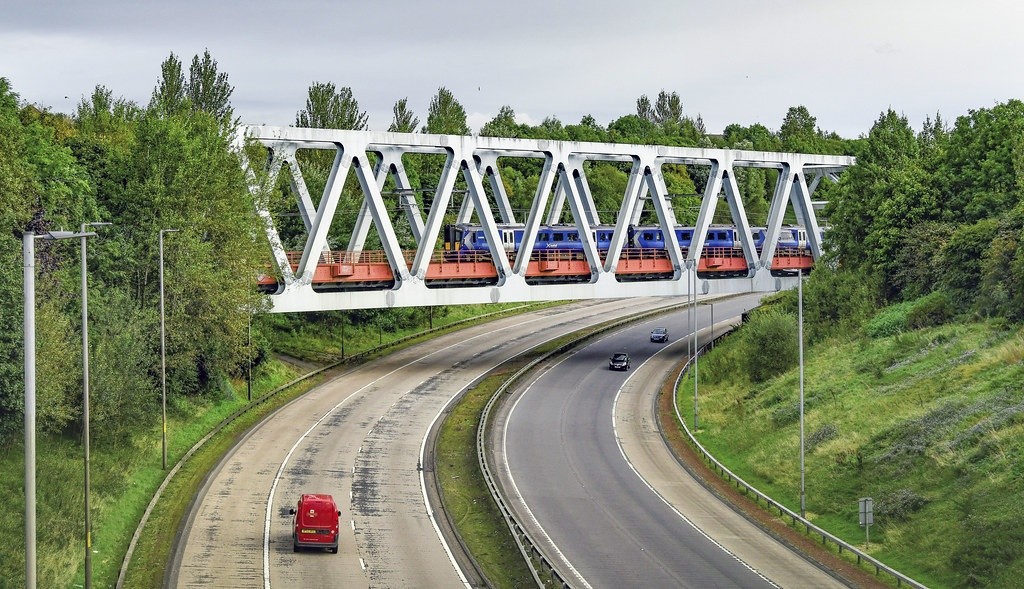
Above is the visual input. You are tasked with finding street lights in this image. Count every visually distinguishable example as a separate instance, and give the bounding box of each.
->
[684,258,699,431]
[159,229,183,470]
[20,221,95,588]
[784,267,806,521]
[79,220,113,589]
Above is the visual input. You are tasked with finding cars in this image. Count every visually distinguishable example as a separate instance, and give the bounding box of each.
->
[610,352,631,372]
[649,327,669,342]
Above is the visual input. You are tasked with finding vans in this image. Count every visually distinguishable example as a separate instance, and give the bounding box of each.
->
[288,492,341,554]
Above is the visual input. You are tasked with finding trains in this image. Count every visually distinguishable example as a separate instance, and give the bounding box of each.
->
[441,222,835,264]
